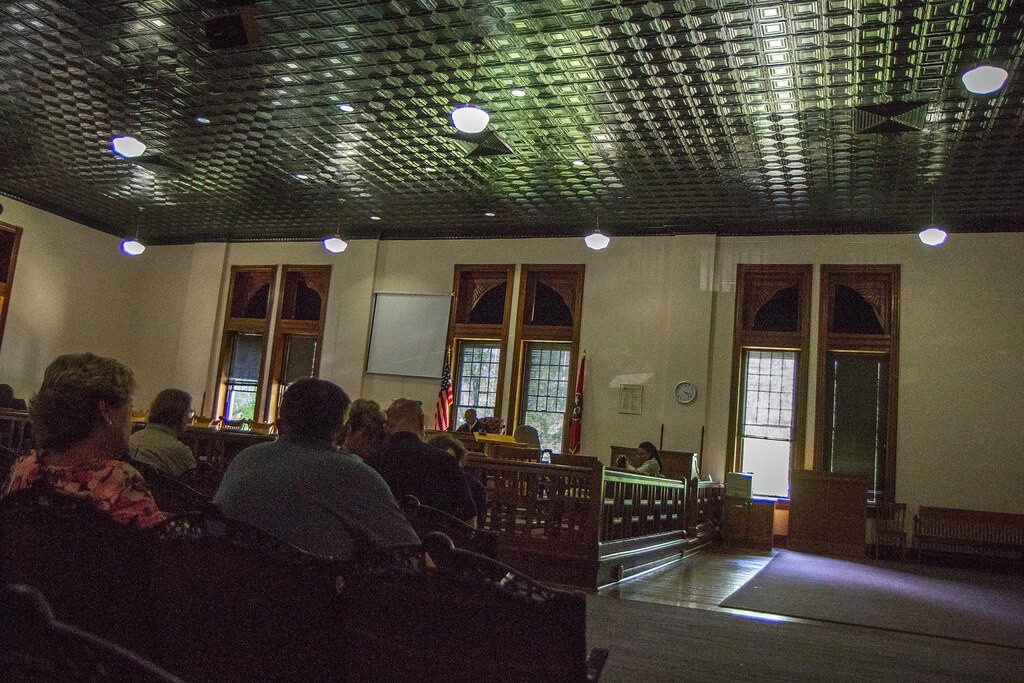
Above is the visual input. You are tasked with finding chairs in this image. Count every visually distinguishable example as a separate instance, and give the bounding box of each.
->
[875,502,908,561]
[0,409,611,683]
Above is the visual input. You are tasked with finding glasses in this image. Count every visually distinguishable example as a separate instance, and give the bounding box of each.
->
[179,408,196,421]
[637,450,649,457]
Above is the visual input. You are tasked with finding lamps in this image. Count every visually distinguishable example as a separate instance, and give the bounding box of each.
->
[323,206,347,252]
[918,178,947,246]
[122,206,145,256]
[960,64,1009,94]
[584,190,610,250]
[112,68,146,158]
[451,40,489,133]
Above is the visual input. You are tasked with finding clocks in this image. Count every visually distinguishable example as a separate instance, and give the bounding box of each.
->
[673,381,697,404]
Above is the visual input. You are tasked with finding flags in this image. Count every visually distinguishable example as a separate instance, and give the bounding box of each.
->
[568,352,585,455]
[435,355,453,433]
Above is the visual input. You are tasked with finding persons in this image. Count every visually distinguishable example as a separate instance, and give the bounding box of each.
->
[616,441,666,478]
[0,351,203,538]
[456,408,491,434]
[213,377,437,568]
[336,398,477,539]
[128,388,197,512]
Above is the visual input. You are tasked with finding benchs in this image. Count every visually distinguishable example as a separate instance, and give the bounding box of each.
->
[913,505,1024,575]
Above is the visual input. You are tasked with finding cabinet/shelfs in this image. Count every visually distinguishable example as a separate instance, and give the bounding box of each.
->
[723,494,776,551]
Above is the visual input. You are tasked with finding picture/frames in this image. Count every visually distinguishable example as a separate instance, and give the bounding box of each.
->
[618,384,644,415]
